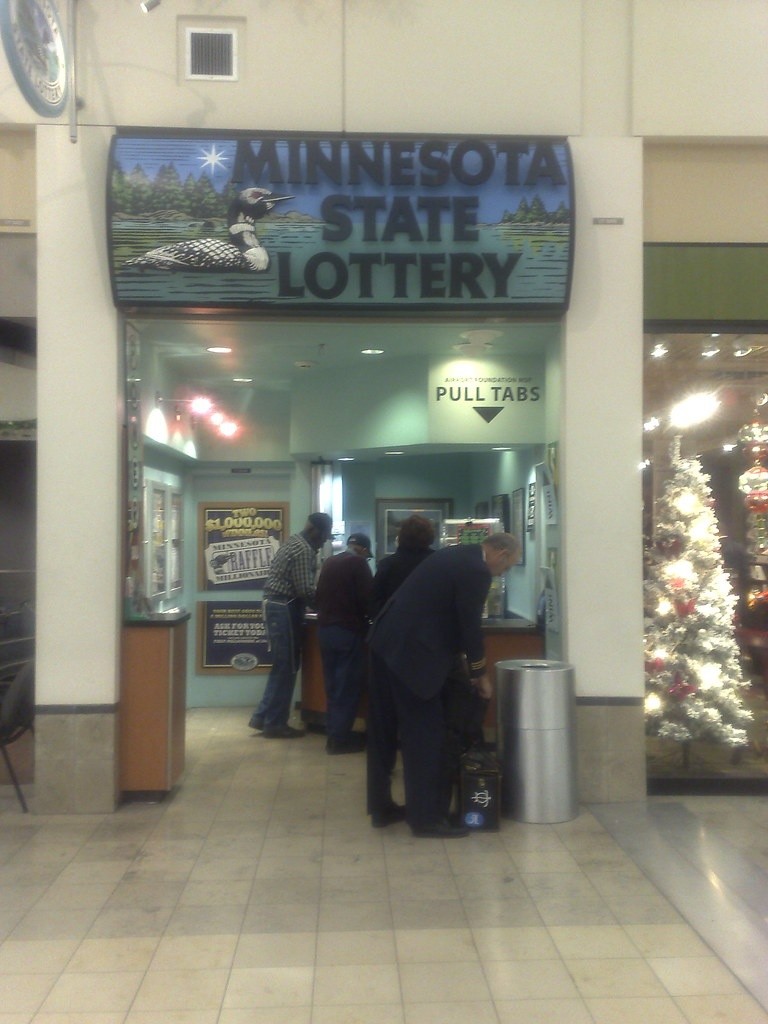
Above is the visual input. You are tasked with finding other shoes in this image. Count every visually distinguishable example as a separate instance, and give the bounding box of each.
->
[326,731,366,754]
[247,719,264,731]
[264,724,304,739]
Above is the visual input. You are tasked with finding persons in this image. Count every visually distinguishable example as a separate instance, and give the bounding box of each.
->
[354,533,521,839]
[368,514,435,625]
[310,532,373,754]
[247,513,332,739]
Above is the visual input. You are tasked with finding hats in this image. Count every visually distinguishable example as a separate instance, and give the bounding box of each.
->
[308,512,335,539]
[346,533,374,558]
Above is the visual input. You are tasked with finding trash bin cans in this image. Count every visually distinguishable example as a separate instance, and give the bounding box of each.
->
[493,657,580,825]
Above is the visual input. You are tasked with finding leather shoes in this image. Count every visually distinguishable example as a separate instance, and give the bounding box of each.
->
[371,802,407,827]
[413,815,469,836]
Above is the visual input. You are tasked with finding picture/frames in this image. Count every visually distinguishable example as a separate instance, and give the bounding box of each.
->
[374,498,453,571]
[476,482,536,566]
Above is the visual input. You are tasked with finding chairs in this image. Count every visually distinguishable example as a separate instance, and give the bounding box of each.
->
[0,655,34,814]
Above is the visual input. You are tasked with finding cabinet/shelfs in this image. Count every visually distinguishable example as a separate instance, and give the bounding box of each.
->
[120,613,190,792]
